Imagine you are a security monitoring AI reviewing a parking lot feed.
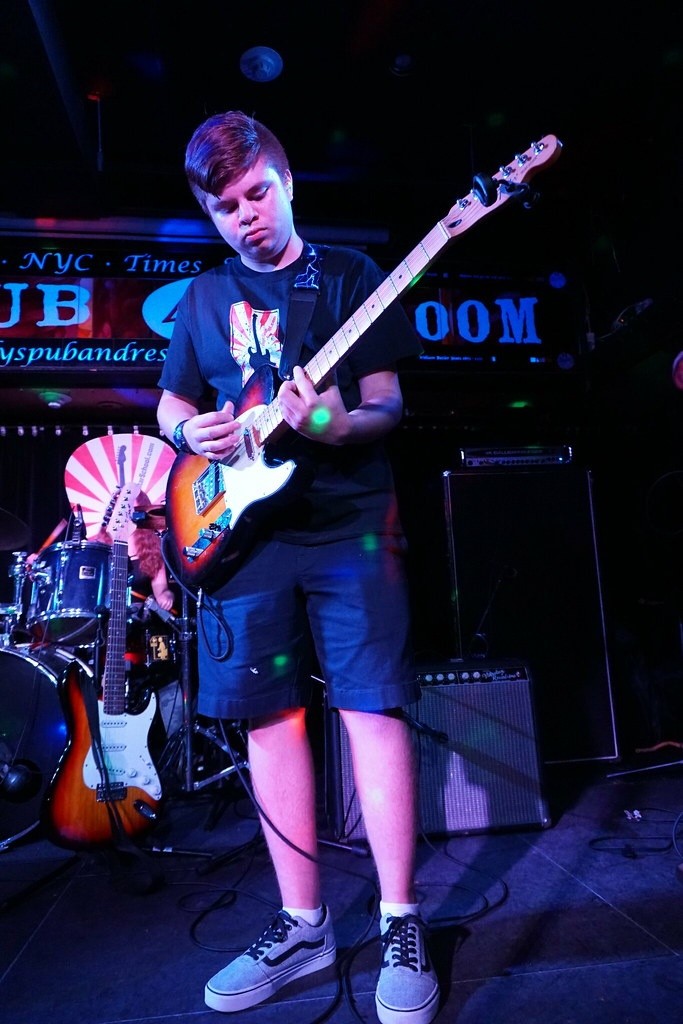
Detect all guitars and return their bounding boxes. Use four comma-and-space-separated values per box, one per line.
35, 482, 166, 855
158, 131, 567, 587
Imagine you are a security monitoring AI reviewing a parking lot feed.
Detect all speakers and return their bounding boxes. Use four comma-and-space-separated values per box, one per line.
442, 468, 619, 765
322, 657, 552, 844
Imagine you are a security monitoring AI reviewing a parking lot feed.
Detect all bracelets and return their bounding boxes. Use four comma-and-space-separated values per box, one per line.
172, 420, 197, 457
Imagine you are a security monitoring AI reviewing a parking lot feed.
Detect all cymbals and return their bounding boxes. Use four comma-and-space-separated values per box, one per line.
0, 508, 32, 551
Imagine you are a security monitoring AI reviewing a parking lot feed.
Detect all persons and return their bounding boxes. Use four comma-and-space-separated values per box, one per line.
156, 112, 441, 1022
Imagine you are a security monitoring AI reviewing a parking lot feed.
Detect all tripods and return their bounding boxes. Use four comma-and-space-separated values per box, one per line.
147, 525, 250, 796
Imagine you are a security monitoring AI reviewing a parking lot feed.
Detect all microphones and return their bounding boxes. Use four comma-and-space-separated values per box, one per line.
77, 504, 86, 539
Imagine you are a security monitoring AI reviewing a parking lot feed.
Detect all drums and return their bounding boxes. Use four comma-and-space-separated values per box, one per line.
0, 641, 98, 844
24, 539, 134, 648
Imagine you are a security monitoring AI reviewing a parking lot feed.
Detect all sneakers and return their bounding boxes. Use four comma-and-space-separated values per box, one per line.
374, 910, 439, 1024
204, 902, 337, 1012
